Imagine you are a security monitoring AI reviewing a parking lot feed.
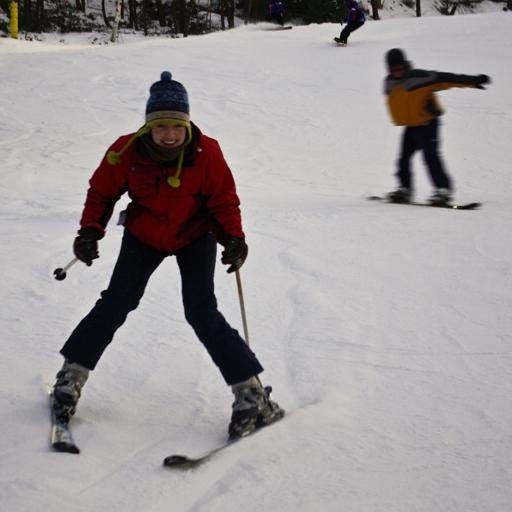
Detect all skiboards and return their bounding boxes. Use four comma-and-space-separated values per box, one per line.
50, 393, 285, 467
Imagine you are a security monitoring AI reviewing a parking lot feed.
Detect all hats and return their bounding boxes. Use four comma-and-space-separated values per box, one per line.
136, 70, 193, 147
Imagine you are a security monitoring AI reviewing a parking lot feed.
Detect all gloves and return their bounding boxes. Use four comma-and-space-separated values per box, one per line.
473, 73, 494, 92
71, 234, 101, 267
220, 239, 248, 274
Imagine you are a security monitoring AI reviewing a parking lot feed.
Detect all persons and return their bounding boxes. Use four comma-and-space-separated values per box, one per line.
385, 48, 491, 203
54, 80, 276, 440
334, 1, 367, 44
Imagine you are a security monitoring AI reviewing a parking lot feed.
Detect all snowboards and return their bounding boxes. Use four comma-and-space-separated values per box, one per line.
334, 37, 346, 46
370, 197, 481, 209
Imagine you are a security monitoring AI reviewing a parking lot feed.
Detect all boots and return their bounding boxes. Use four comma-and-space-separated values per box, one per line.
388, 181, 414, 201
52, 360, 90, 405
426, 187, 456, 202
225, 384, 282, 437
333, 37, 349, 44
